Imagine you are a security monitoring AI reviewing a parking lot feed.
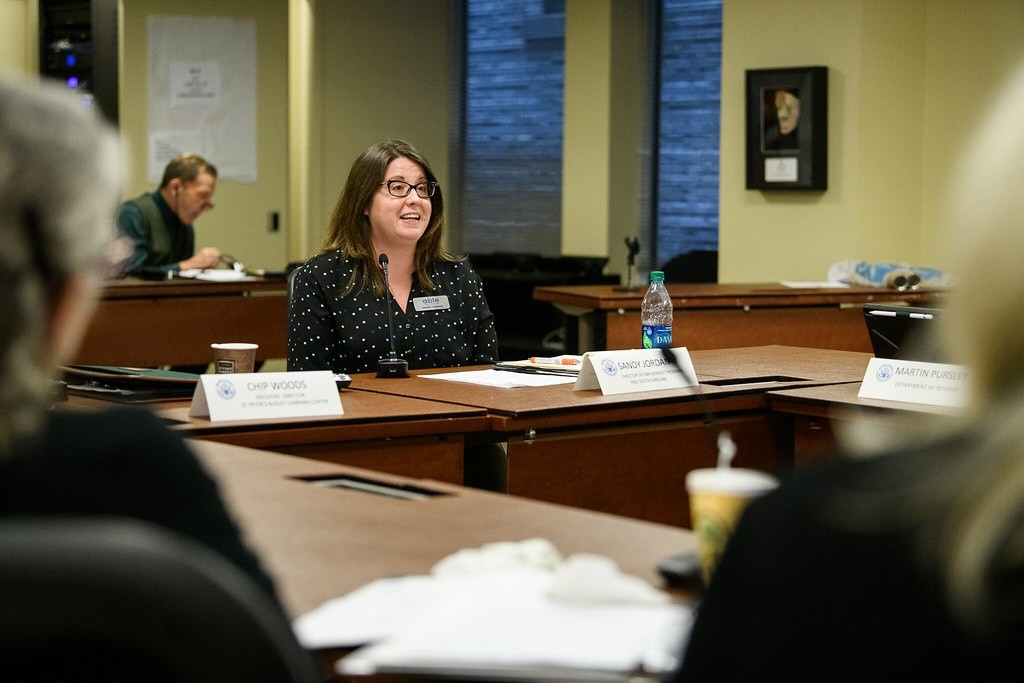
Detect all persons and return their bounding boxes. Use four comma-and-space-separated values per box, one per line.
0, 74, 307, 683
287, 139, 500, 374
667, 67, 1024, 683
118, 153, 222, 273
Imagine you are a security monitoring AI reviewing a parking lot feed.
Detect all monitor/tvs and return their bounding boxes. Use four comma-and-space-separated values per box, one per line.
862, 304, 943, 364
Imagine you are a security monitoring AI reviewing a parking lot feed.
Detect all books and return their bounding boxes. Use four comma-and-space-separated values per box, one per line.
494, 354, 584, 378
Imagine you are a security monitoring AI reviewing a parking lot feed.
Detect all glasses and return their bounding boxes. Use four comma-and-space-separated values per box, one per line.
380, 180, 438, 199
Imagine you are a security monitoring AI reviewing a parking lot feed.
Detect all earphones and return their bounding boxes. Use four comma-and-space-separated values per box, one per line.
174, 187, 178, 192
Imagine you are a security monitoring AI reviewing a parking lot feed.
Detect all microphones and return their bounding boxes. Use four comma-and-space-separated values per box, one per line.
661, 346, 717, 446
374, 253, 411, 378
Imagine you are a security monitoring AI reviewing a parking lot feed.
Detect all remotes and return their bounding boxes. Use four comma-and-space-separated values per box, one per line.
333, 373, 352, 387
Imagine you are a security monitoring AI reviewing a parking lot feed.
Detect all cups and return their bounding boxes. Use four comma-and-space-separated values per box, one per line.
688, 470, 777, 585
212, 343, 258, 373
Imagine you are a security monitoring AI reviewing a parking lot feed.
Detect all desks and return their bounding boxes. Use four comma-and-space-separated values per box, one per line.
63, 343, 986, 539
534, 283, 956, 371
66, 274, 313, 370
181, 436, 762, 683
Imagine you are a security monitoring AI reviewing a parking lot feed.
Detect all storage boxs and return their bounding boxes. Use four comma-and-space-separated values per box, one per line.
745, 66, 829, 192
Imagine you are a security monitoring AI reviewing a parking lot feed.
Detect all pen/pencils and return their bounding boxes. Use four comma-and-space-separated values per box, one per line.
527, 356, 581, 365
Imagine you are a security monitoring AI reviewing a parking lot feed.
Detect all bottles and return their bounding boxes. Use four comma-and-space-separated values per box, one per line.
641, 271, 672, 349
